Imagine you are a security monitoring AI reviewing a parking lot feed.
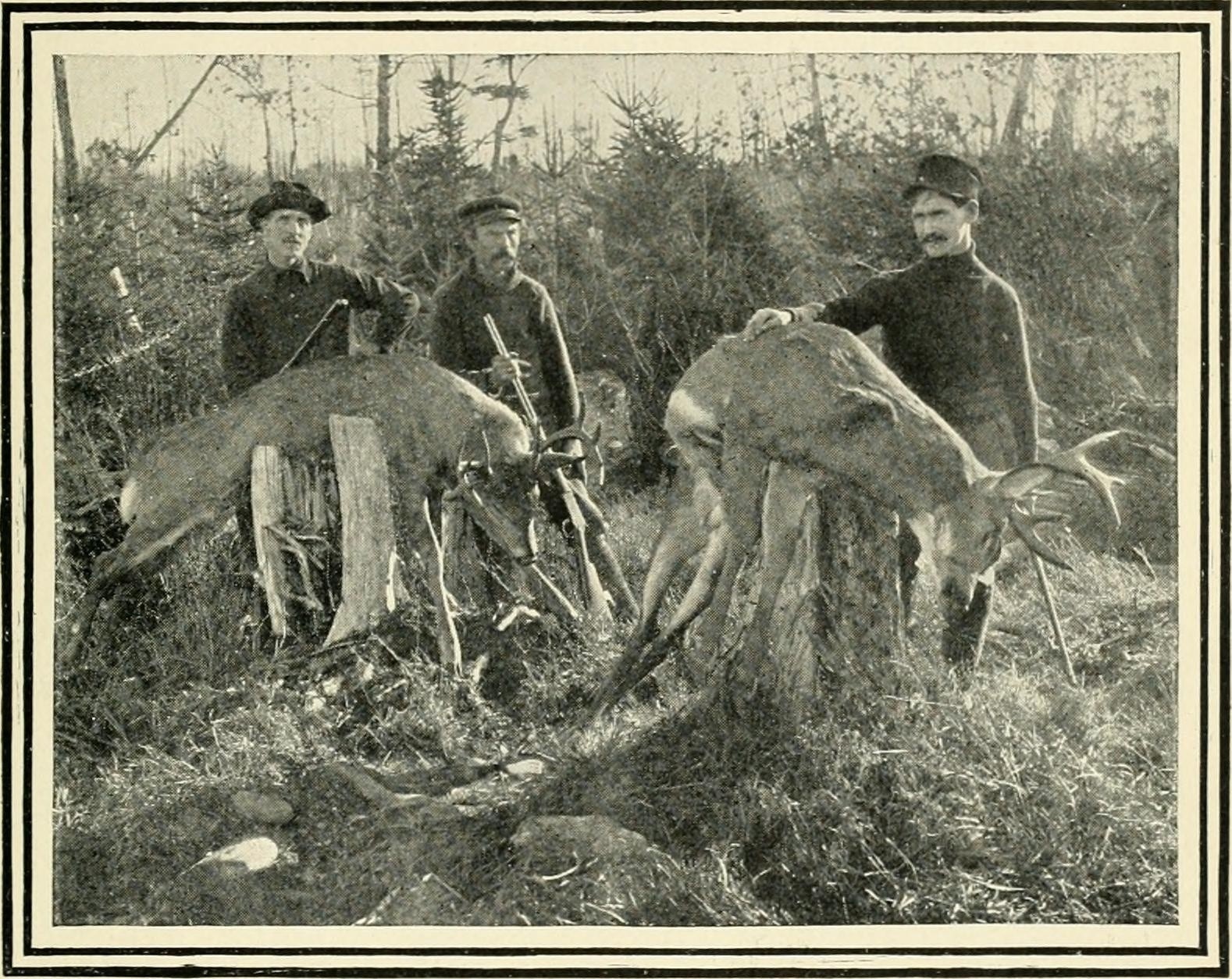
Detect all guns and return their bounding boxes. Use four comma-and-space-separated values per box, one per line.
280, 296, 353, 373
994, 419, 1079, 692
481, 309, 642, 623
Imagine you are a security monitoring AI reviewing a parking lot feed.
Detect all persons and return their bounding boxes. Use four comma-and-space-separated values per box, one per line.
429, 197, 586, 609
742, 155, 1038, 690
221, 179, 421, 648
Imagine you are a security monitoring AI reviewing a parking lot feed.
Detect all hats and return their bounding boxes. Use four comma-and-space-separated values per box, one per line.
248, 179, 333, 230
901, 153, 983, 203
458, 194, 523, 230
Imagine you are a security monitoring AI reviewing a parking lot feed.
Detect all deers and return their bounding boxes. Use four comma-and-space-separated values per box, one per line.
586, 318, 1177, 739
60, 355, 606, 691
